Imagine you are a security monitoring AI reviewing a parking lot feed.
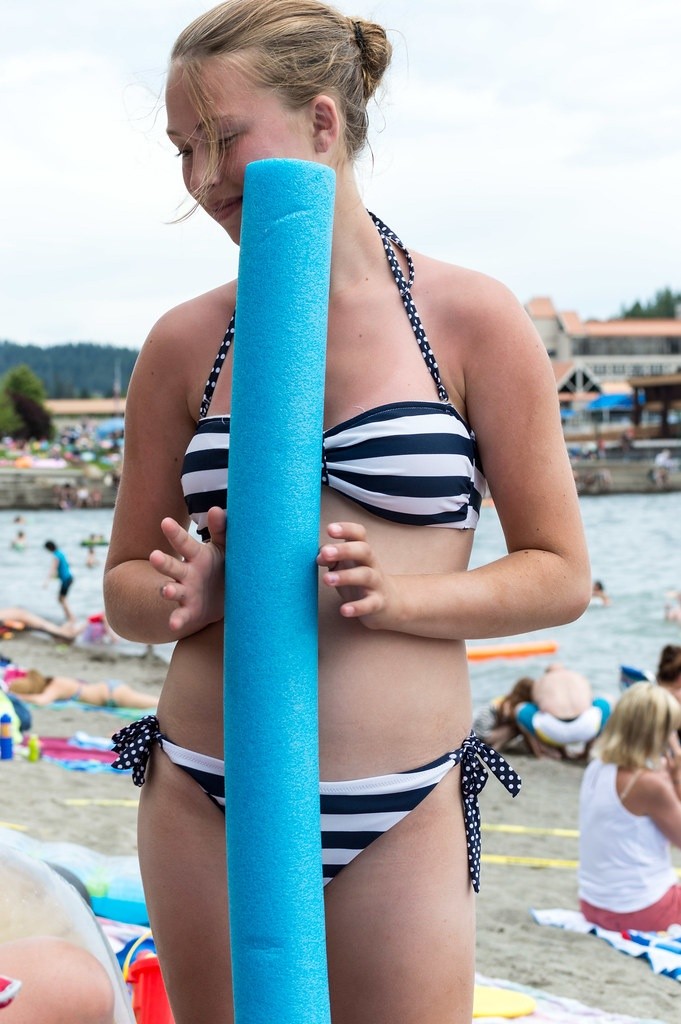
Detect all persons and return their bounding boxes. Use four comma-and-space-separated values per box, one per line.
102, 1, 593, 1023
566, 429, 675, 493
0, 512, 159, 711
0, 935, 115, 1024
0, 417, 121, 514
470, 576, 680, 933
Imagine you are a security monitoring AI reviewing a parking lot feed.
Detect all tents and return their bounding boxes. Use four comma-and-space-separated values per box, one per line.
585, 392, 645, 430
